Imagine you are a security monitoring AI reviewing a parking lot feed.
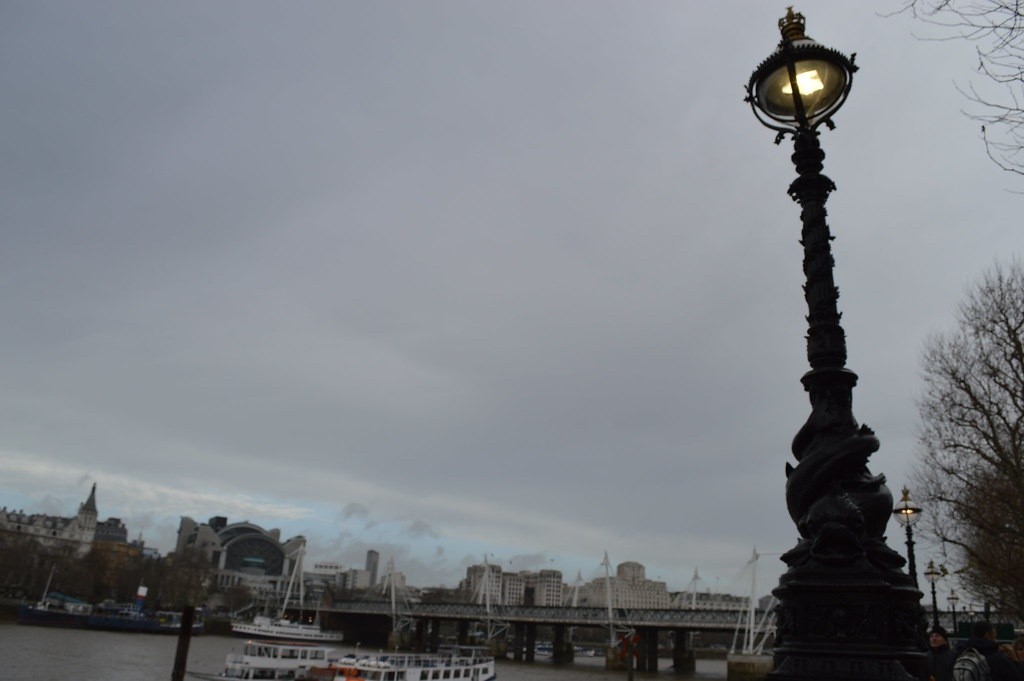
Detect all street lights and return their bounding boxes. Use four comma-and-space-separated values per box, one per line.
925, 559, 945, 635
743, 5, 933, 681
946, 588, 960, 637
891, 484, 923, 592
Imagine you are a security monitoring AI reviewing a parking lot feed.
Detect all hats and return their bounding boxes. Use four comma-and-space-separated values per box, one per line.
929, 626, 948, 639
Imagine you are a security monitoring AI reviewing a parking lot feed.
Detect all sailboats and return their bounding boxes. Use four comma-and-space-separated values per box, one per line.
230, 542, 344, 644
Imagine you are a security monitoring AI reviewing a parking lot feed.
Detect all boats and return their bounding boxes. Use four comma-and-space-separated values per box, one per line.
324, 642, 497, 681
20, 560, 163, 631
183, 638, 344, 681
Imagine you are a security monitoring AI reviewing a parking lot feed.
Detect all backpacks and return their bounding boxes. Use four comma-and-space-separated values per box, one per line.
952, 646, 997, 681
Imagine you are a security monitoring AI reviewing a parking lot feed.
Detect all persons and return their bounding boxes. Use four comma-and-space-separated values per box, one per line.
946, 621, 1024, 681
929, 627, 953, 681
